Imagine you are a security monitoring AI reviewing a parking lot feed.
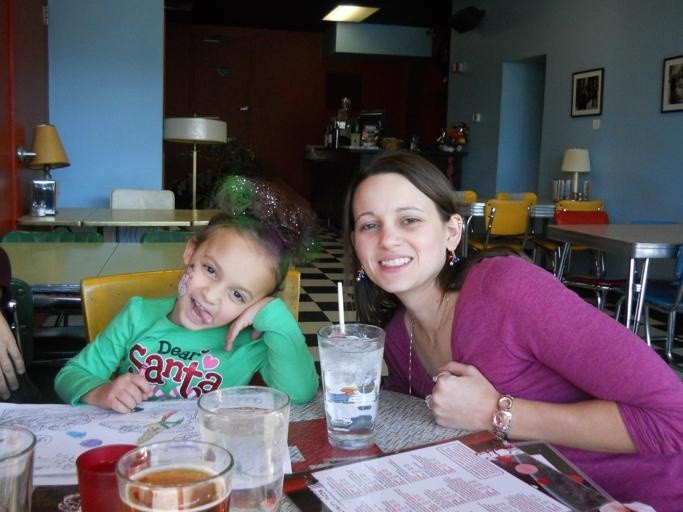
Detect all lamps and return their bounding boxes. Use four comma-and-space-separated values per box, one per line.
556, 145, 594, 198
23, 119, 72, 182
160, 111, 232, 209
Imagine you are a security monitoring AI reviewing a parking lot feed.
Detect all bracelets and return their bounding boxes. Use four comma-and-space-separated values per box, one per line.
491, 394, 514, 441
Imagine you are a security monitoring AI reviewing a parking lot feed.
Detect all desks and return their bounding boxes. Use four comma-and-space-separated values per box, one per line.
303, 145, 474, 230
1, 390, 629, 511
0, 239, 191, 288
2, 207, 234, 244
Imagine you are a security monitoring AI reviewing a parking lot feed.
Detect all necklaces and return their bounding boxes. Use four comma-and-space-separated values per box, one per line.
408, 290, 451, 395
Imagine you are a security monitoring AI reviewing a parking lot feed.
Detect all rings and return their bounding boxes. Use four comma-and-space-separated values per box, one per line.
425, 393, 432, 409
432, 371, 450, 383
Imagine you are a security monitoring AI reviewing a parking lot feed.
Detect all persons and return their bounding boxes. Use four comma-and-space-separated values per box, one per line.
0, 247, 27, 402
342, 149, 682, 512
53, 173, 321, 414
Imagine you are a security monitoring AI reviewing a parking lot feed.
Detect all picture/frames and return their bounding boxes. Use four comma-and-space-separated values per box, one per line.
659, 53, 683, 118
567, 66, 607, 120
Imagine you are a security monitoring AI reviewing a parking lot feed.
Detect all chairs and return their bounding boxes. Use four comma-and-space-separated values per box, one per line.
3, 278, 82, 401
451, 188, 682, 359
110, 188, 175, 209
81, 267, 302, 343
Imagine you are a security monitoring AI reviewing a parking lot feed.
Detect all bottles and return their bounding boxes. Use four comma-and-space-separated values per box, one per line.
552, 177, 570, 201
322, 116, 339, 148
578, 178, 590, 201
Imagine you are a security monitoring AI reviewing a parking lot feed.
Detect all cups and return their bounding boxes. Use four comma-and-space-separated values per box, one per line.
195, 385, 291, 512
0, 424, 36, 511
73, 442, 154, 512
317, 324, 387, 448
118, 442, 234, 512
